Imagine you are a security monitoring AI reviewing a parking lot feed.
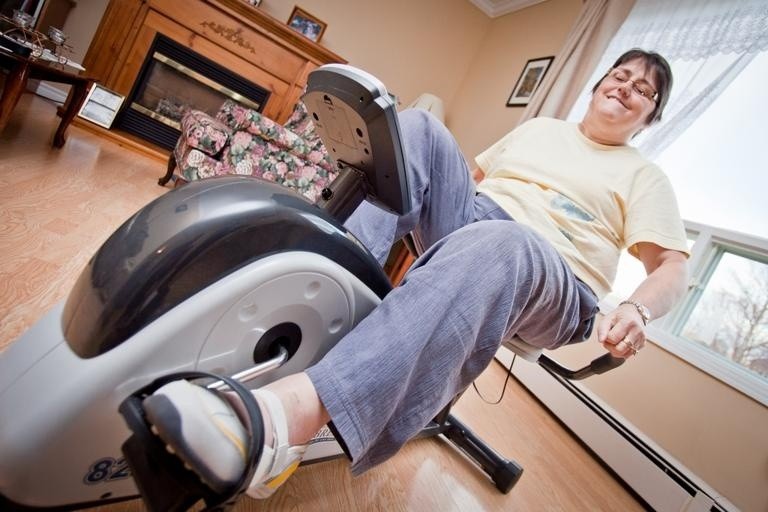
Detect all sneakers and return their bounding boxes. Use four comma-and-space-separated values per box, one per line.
137, 374, 312, 502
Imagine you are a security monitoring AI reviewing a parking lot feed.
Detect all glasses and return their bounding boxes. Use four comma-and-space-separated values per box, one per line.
606, 65, 656, 100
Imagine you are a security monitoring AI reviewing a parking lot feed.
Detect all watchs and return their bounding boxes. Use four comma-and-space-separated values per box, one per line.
618, 300, 651, 326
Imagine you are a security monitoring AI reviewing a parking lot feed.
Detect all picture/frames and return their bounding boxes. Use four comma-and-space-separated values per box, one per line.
285, 5, 329, 42
506, 55, 556, 108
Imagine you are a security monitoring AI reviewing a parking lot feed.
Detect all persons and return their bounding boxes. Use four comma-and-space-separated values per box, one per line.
139, 44, 691, 500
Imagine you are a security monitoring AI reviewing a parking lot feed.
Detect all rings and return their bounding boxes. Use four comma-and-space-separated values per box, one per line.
622, 338, 633, 348
629, 347, 639, 356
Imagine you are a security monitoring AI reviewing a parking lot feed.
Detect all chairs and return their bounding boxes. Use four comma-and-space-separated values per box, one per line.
158, 81, 339, 202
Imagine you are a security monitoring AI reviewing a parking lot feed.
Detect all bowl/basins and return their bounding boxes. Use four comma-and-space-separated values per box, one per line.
13, 8, 35, 29
47, 25, 65, 46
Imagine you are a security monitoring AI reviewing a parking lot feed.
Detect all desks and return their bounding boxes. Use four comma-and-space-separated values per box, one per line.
0, 37, 102, 149
